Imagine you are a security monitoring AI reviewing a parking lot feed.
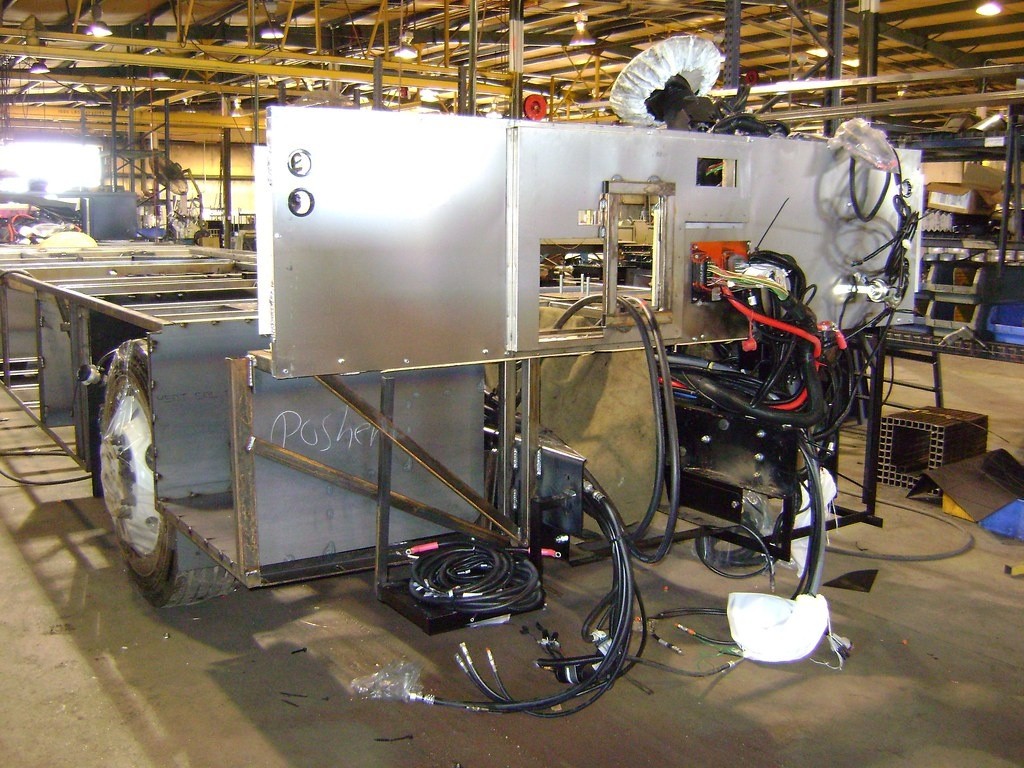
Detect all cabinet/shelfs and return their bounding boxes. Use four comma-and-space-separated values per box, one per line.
884, 136, 1024, 364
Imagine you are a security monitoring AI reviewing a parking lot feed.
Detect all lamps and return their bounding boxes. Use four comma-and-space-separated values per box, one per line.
182, 98, 196, 114
151, 72, 170, 82
84, 4, 113, 37
793, 54, 809, 82
713, 31, 726, 63
30, 58, 50, 74
569, 21, 596, 46
260, 1, 284, 39
230, 98, 246, 117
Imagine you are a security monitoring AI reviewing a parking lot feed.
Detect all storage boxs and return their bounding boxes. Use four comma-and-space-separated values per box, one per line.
926, 163, 1005, 215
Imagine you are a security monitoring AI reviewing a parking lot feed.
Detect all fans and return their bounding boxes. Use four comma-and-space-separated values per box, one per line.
149, 155, 204, 229
